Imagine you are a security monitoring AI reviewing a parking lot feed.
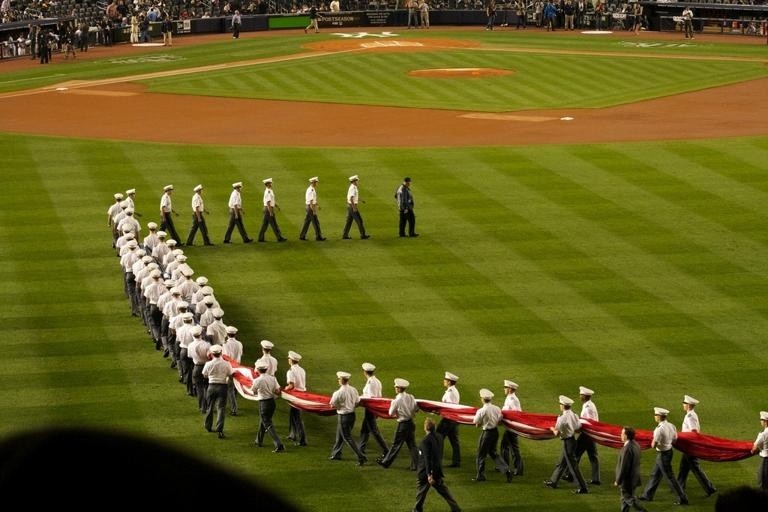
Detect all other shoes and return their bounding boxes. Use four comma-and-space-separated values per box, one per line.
446, 462, 459, 468
543, 480, 557, 489
512, 472, 523, 475
495, 466, 501, 472
704, 489, 717, 498
586, 479, 600, 485
572, 488, 588, 495
255, 436, 308, 453
472, 475, 485, 483
327, 443, 419, 472
637, 495, 653, 501
673, 500, 688, 505
560, 475, 574, 483
505, 471, 513, 483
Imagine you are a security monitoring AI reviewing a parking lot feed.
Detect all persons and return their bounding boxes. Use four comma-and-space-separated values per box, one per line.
752, 410, 768, 490
682, 7, 694, 40
434, 370, 461, 468
542, 386, 601, 494
107, 181, 253, 442
614, 395, 716, 510
253, 339, 308, 452
342, 174, 371, 240
715, 486, 767, 510
178, 1, 340, 39
486, 0, 644, 34
258, 177, 288, 243
299, 176, 327, 241
396, 177, 418, 237
413, 418, 462, 511
329, 362, 390, 465
405, 0, 430, 29
1, 1, 174, 64
375, 377, 420, 471
472, 380, 523, 483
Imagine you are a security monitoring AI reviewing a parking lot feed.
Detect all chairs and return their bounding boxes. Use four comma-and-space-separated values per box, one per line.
1, 1, 223, 48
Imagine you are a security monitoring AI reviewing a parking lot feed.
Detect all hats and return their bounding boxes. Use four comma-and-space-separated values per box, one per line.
255, 358, 269, 370
479, 388, 494, 401
444, 371, 459, 382
262, 177, 273, 185
260, 339, 275, 350
653, 406, 670, 417
681, 394, 700, 405
760, 410, 768, 421
558, 394, 575, 407
578, 386, 595, 397
308, 176, 318, 183
348, 174, 360, 182
287, 350, 302, 362
393, 377, 410, 389
503, 379, 519, 390
336, 370, 352, 380
361, 362, 376, 372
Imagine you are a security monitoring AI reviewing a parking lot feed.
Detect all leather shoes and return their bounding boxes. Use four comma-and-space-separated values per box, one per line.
257, 233, 419, 242
114, 179, 253, 439
